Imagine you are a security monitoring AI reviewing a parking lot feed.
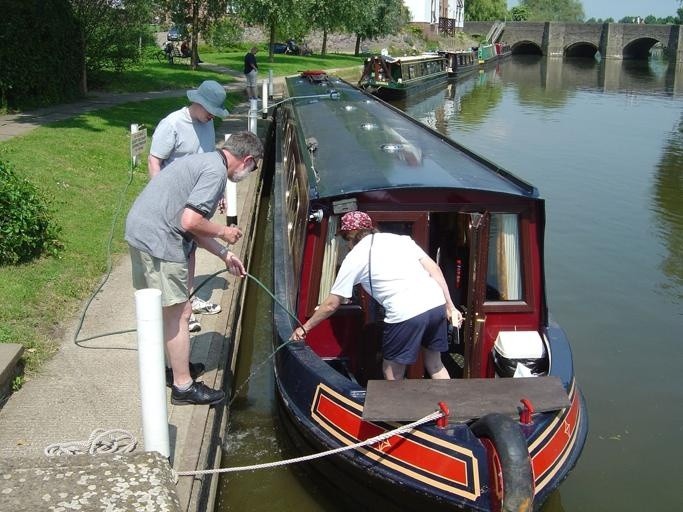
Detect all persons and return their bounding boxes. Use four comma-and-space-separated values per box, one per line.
146, 79, 228, 331
289, 211, 462, 380
125, 131, 265, 404
181, 40, 204, 63
244, 47, 261, 100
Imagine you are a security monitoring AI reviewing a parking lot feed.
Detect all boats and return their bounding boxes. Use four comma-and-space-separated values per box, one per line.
275, 72, 590, 512
357, 40, 513, 98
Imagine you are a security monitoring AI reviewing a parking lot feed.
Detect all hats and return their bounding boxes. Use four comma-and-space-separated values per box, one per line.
334, 211, 374, 238
186, 80, 230, 119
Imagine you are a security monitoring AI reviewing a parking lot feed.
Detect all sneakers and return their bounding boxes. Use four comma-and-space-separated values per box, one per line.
191, 296, 223, 315
188, 313, 202, 332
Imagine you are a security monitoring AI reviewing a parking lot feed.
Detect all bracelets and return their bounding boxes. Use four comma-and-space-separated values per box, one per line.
220, 248, 228, 255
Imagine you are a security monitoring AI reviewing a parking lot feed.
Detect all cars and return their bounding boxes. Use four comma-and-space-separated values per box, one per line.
273, 43, 304, 54
167, 25, 189, 41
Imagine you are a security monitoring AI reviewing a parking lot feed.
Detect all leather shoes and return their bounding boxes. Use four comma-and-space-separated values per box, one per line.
165, 361, 205, 386
171, 383, 225, 405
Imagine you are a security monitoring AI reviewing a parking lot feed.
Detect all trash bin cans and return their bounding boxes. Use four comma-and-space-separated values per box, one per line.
492, 330, 549, 378
